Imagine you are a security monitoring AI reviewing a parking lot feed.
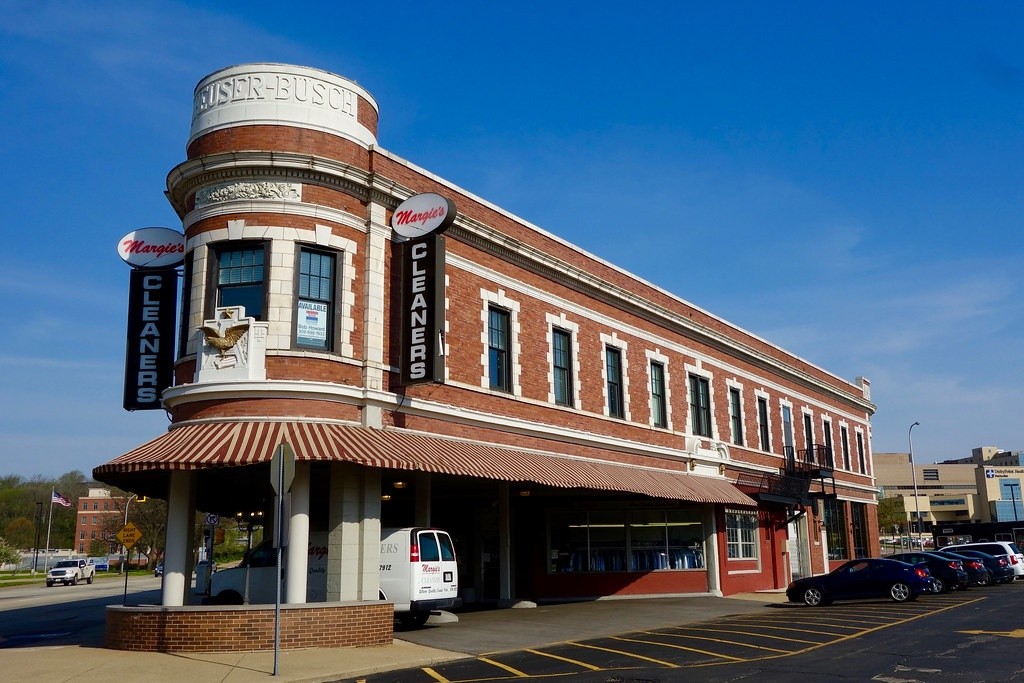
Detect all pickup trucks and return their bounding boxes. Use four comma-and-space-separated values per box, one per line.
46, 559, 96, 585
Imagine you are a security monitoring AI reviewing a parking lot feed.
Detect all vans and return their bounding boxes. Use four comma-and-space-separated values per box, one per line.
202, 527, 464, 630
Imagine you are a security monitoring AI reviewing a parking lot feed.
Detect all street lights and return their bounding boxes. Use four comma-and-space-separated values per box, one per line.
235, 510, 265, 604
909, 421, 924, 552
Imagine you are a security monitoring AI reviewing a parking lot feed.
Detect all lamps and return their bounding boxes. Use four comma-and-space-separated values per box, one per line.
691, 458, 697, 467
520, 489, 530, 496
393, 481, 407, 488
381, 494, 391, 500
818, 521, 827, 527
720, 463, 726, 472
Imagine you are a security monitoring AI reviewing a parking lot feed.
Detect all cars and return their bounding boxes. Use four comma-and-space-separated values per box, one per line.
155, 556, 165, 577
885, 553, 965, 597
880, 537, 934, 547
195, 559, 216, 573
933, 552, 988, 589
955, 550, 1010, 586
785, 558, 931, 605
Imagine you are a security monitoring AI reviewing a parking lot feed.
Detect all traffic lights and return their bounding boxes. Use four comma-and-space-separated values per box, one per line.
137, 494, 146, 503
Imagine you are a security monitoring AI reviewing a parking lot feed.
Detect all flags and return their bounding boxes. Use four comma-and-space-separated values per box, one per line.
52, 490, 72, 507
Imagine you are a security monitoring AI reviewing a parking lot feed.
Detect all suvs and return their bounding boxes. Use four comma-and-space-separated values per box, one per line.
940, 542, 1024, 582
